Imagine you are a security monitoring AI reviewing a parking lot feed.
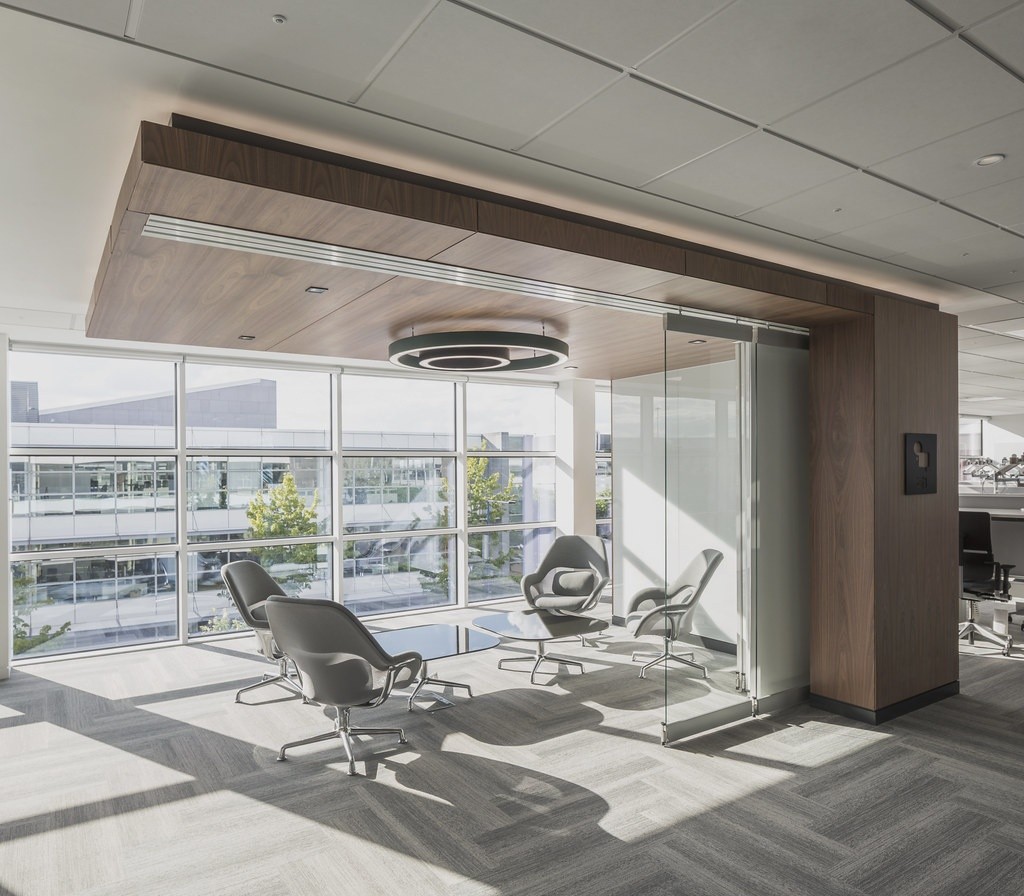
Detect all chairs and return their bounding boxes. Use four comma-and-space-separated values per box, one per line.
521, 535, 610, 646
221, 560, 309, 705
265, 596, 422, 775
959, 511, 1016, 656
626, 549, 724, 679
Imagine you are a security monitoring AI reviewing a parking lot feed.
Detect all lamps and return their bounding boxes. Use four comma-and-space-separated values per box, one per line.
388, 326, 569, 373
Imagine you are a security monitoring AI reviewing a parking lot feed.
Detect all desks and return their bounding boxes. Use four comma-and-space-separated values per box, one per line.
472, 609, 610, 684
370, 623, 500, 712
